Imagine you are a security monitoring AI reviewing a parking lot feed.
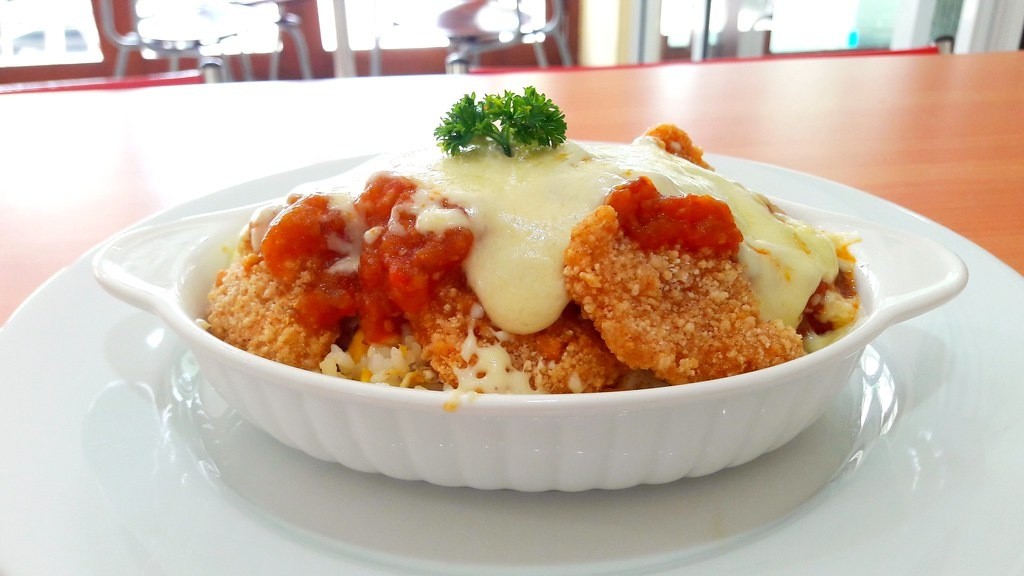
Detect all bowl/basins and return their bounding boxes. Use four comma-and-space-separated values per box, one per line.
91, 193, 970, 494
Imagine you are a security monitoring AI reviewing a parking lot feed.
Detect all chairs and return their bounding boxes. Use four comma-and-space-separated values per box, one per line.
99, 0, 577, 81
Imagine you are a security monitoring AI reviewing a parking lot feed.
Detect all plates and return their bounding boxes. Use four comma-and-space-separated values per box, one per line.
0, 139, 1024, 576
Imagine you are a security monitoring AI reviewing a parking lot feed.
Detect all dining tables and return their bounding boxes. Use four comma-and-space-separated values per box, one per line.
0, 47, 1024, 328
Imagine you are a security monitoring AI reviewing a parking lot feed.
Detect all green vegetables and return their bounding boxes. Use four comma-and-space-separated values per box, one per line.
432, 87, 569, 157
